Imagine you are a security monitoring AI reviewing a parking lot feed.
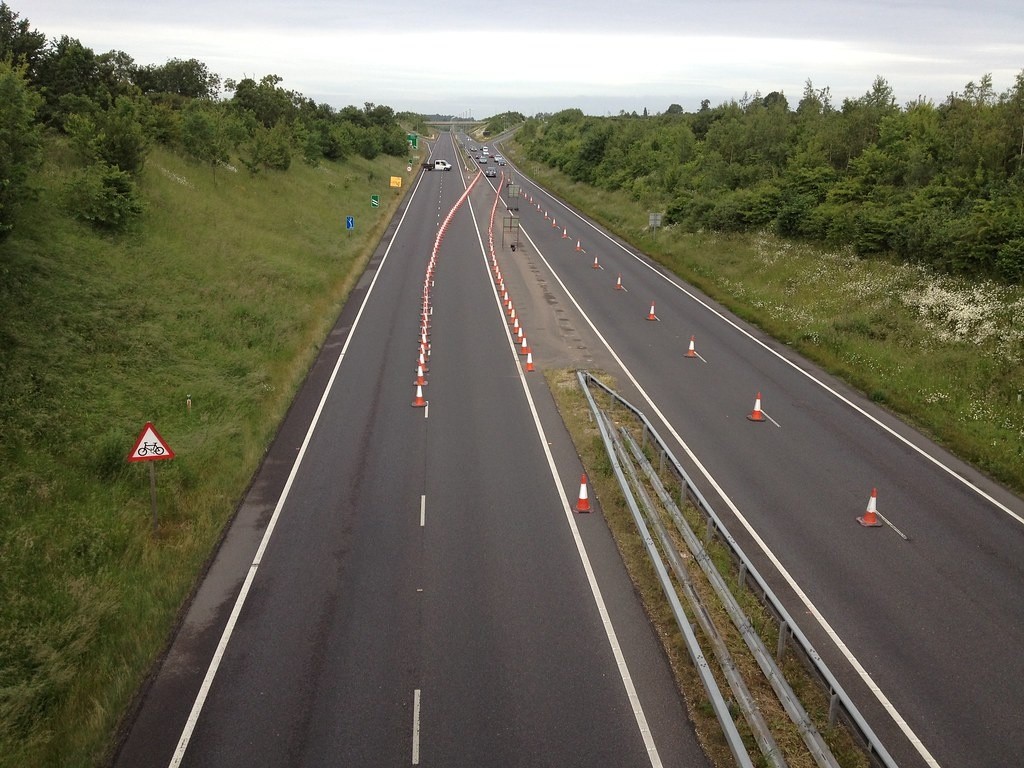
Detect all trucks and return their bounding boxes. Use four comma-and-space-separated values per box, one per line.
420, 160, 453, 171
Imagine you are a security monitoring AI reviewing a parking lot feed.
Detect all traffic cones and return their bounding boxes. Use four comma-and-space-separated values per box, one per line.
591, 256, 601, 269
415, 347, 430, 374
413, 363, 429, 386
646, 300, 658, 321
416, 339, 430, 362
856, 488, 883, 527
524, 347, 536, 371
747, 392, 766, 422
505, 296, 514, 316
507, 305, 517, 324
517, 331, 532, 355
414, 168, 568, 329
682, 335, 697, 358
572, 474, 595, 514
410, 376, 428, 408
417, 328, 431, 351
500, 284, 506, 297
574, 239, 581, 252
502, 288, 510, 307
612, 272, 624, 291
513, 323, 526, 344
512, 313, 520, 335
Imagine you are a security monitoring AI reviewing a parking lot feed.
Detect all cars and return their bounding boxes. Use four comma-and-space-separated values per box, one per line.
470, 146, 507, 167
484, 166, 497, 177
